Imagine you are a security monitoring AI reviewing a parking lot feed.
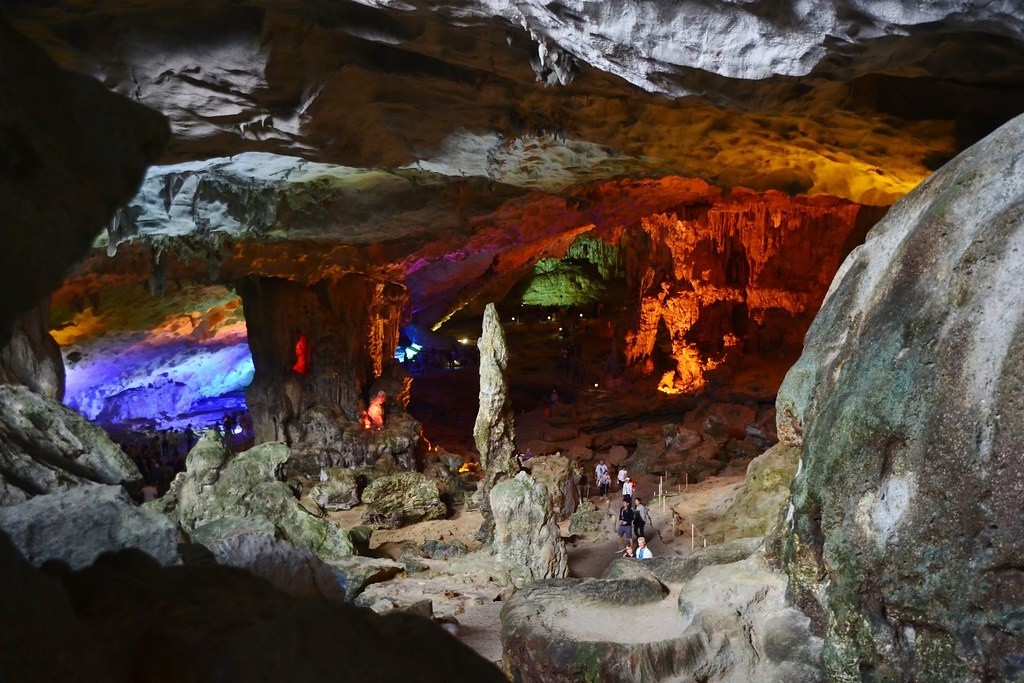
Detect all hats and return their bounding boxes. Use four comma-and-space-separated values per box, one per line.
625, 478, 631, 481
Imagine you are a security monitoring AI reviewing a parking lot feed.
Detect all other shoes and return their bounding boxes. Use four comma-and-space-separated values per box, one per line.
614, 546, 626, 553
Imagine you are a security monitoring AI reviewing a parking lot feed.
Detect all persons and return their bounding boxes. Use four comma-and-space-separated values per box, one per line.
636, 537, 652, 558
576, 456, 582, 466
119, 426, 179, 474
517, 305, 581, 357
614, 499, 634, 553
623, 476, 635, 501
214, 411, 252, 441
458, 457, 478, 473
617, 465, 628, 493
523, 448, 532, 460
599, 471, 612, 502
550, 390, 559, 413
184, 424, 198, 451
624, 545, 636, 558
633, 497, 647, 538
403, 342, 476, 369
595, 460, 607, 487
